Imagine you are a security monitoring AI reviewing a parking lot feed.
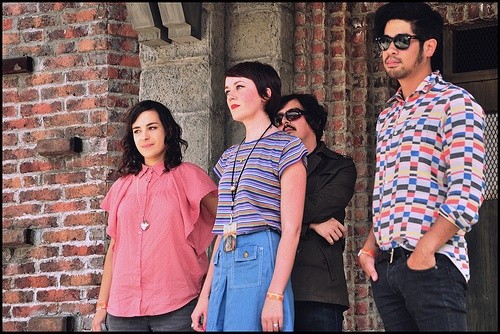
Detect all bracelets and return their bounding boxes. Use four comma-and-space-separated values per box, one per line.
266, 292, 284, 301
96, 305, 107, 310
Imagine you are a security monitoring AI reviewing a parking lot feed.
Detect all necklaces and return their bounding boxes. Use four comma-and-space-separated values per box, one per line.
136, 174, 164, 232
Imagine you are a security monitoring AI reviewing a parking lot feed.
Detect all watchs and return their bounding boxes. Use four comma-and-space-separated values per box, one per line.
357, 248, 376, 258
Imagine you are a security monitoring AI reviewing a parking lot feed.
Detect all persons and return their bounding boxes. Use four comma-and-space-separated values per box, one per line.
191, 60, 358, 333
357, 0, 487, 334
90, 99, 218, 333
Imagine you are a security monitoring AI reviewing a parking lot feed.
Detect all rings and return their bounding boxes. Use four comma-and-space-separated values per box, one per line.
91, 329, 93, 332
273, 324, 278, 327
190, 323, 194, 328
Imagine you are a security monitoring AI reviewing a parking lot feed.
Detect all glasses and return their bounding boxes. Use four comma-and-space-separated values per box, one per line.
374, 34, 419, 50
274, 108, 306, 127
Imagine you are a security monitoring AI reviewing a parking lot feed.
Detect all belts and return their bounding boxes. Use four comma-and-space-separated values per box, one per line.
378, 247, 413, 263
